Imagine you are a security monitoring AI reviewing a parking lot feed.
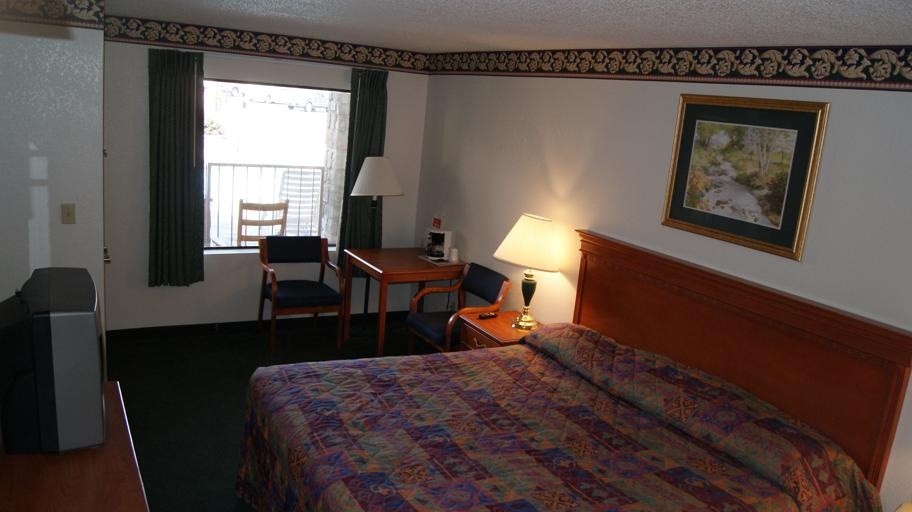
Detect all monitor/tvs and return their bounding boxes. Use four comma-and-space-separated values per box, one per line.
0, 267, 107, 455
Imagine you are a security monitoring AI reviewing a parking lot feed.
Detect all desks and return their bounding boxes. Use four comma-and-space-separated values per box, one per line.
0, 380, 151, 512
343, 248, 466, 357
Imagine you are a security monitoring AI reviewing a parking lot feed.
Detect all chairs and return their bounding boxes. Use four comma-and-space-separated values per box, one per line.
258, 236, 346, 358
212, 199, 289, 246
405, 262, 512, 356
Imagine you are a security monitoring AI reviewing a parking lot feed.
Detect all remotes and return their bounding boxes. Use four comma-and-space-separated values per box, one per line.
480, 312, 496, 318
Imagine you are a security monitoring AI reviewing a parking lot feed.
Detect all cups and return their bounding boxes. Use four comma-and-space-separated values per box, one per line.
449, 248, 459, 263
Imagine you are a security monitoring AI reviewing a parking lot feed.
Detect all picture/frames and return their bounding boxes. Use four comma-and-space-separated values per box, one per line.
660, 93, 832, 263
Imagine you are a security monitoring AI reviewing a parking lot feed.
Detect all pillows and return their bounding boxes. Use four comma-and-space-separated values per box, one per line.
518, 323, 866, 511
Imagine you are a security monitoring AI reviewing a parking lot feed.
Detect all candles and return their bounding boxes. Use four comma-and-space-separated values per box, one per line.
449, 245, 459, 263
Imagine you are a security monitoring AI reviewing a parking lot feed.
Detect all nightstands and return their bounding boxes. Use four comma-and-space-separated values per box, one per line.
457, 310, 546, 351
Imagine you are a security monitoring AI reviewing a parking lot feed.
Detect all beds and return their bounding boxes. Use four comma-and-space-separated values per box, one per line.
235, 229, 912, 512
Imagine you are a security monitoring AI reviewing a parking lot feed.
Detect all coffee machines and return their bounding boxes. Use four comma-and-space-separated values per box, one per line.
425, 230, 453, 260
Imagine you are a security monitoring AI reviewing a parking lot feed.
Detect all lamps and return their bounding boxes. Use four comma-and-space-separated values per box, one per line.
492, 213, 561, 330
350, 156, 406, 320
